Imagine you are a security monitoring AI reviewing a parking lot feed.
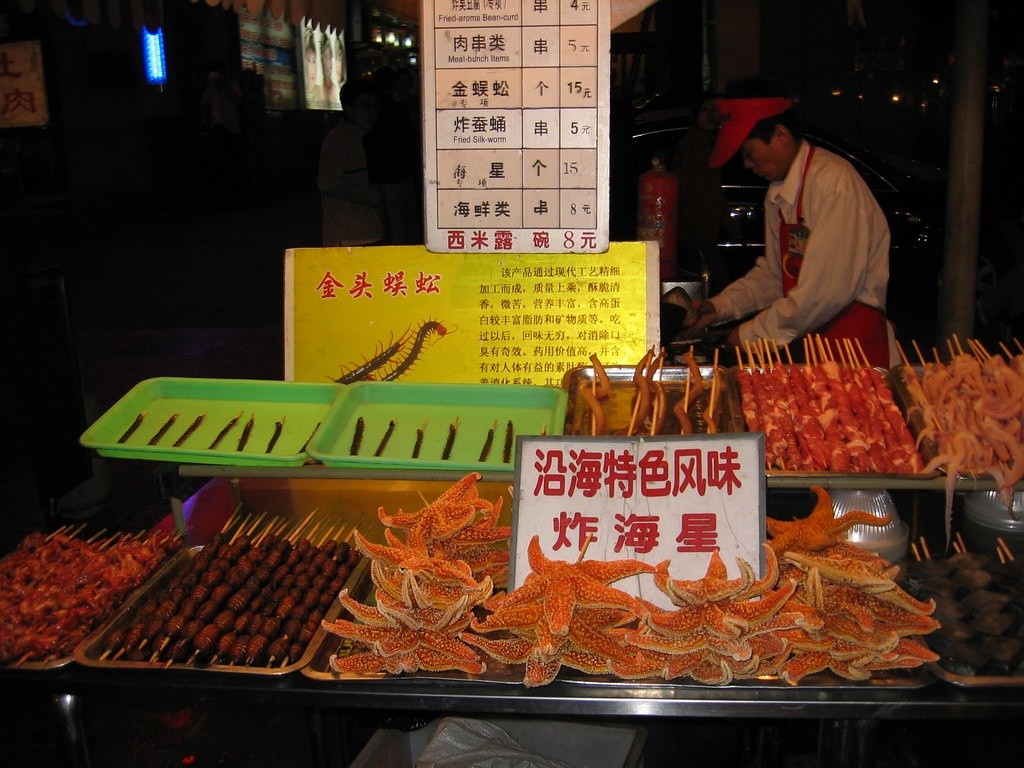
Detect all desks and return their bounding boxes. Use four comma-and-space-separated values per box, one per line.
0, 482, 1024, 768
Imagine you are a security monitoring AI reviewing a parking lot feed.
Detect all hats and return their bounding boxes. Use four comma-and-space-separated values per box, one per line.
710, 98, 791, 168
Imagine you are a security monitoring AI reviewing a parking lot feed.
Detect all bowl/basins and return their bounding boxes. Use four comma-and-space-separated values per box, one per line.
827, 490, 911, 563
963, 488, 1024, 532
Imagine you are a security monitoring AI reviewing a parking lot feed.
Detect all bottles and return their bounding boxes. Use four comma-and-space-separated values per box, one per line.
639, 156, 676, 280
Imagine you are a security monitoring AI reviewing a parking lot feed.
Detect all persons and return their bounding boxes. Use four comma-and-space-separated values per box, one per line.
364, 64, 422, 246
201, 60, 246, 207
683, 97, 890, 367
677, 90, 731, 293
318, 80, 389, 245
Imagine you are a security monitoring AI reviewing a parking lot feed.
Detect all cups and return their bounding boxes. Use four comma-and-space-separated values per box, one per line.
662, 287, 702, 332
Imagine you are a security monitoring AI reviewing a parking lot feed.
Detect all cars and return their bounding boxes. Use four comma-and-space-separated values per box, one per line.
627, 117, 1005, 310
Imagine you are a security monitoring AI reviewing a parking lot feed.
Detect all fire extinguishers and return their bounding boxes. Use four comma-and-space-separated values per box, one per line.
636, 151, 679, 283
